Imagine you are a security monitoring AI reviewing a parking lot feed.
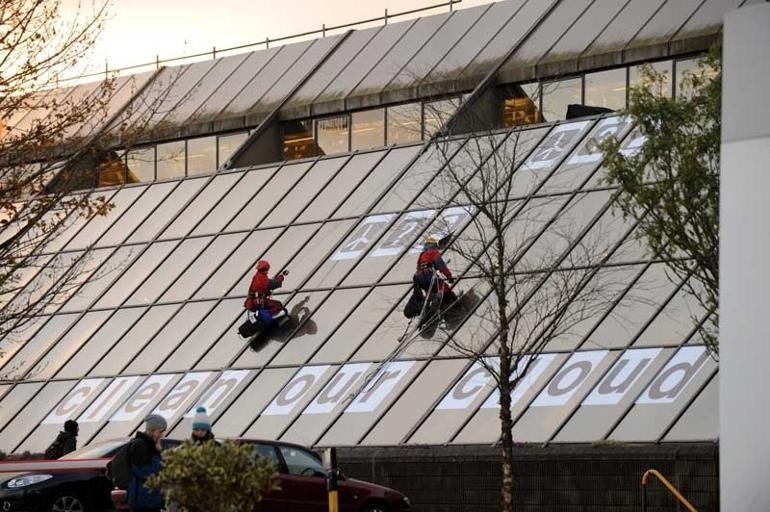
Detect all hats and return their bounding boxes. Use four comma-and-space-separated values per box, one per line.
143, 412, 169, 430
191, 406, 212, 430
63, 419, 77, 431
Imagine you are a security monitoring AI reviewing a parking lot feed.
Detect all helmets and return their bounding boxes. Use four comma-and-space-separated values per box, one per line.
423, 237, 438, 244
255, 259, 271, 270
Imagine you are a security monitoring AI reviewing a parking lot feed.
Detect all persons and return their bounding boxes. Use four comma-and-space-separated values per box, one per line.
183, 406, 222, 450
45, 419, 80, 462
240, 256, 290, 328
416, 236, 455, 300
123, 412, 169, 510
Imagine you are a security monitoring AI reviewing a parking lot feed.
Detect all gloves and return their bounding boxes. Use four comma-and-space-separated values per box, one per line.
448, 278, 454, 283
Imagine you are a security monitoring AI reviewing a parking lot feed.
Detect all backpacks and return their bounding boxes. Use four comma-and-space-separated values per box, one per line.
44, 435, 73, 458
104, 437, 149, 490
